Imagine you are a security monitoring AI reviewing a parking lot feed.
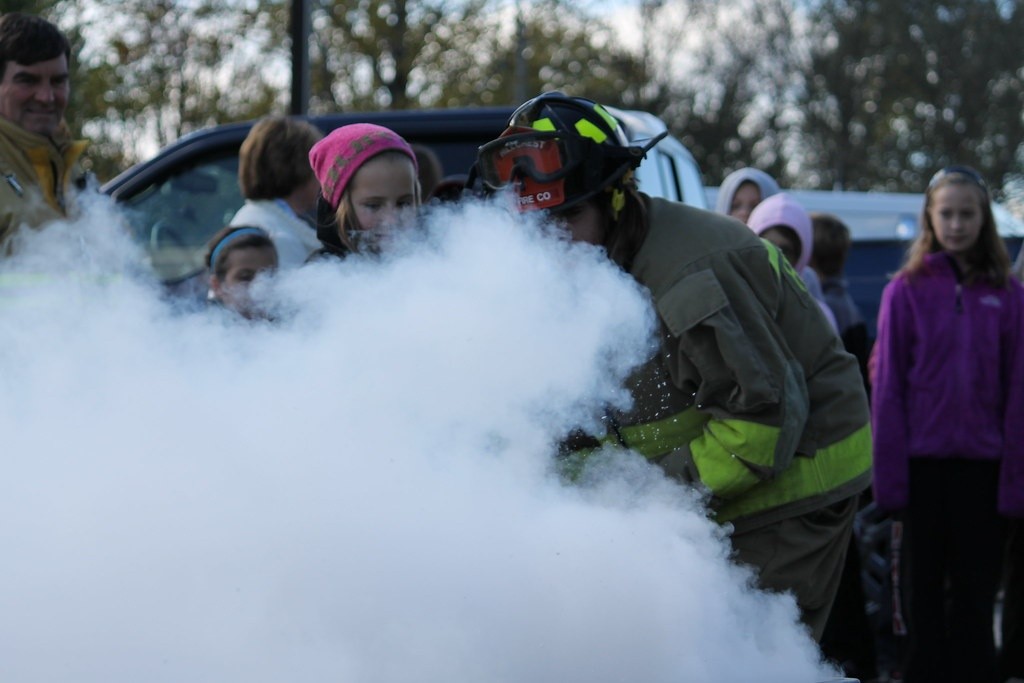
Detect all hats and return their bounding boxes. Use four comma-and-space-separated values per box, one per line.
716, 167, 779, 215
748, 191, 811, 274
307, 123, 418, 207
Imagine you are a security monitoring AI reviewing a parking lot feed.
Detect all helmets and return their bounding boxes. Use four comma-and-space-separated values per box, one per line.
489, 91, 639, 220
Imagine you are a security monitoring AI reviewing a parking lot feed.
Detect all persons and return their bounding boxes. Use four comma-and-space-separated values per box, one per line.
714, 167, 899, 683
230, 118, 326, 264
305, 123, 424, 271
194, 227, 279, 318
468, 90, 872, 648
0, 16, 97, 257
872, 167, 1024, 683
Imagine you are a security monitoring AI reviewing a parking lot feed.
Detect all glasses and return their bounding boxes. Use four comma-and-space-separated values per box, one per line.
476, 131, 596, 191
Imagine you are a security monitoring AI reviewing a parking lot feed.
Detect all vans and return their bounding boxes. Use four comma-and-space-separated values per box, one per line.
94, 102, 710, 315
705, 187, 1024, 385
